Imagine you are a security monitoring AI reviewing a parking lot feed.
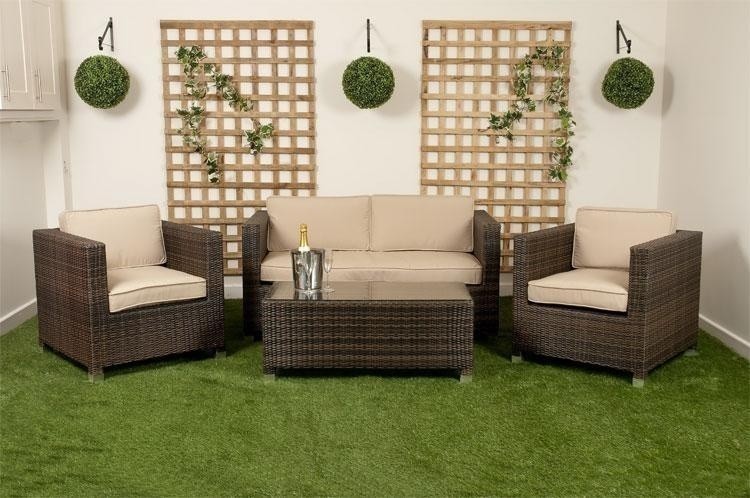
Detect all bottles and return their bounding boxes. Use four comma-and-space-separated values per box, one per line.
298, 223, 311, 251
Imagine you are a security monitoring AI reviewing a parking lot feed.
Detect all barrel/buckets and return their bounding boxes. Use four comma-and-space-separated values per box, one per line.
292, 246, 324, 289
293, 288, 323, 301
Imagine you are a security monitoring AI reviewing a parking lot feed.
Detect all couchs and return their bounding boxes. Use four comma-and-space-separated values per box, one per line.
240, 195, 502, 352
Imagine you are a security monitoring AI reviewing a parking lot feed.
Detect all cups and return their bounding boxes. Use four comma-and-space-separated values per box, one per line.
320, 247, 335, 293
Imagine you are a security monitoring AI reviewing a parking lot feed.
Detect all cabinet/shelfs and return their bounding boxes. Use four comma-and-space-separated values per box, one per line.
0, 0, 67, 121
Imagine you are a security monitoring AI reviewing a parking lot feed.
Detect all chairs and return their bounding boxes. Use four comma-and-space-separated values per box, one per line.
32, 204, 227, 384
511, 207, 703, 388
